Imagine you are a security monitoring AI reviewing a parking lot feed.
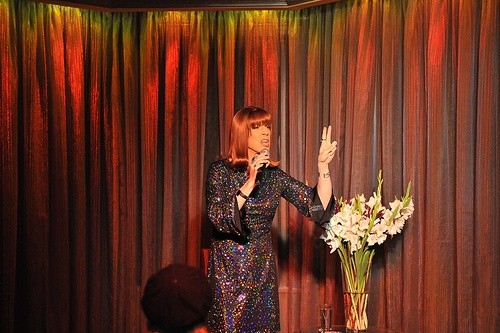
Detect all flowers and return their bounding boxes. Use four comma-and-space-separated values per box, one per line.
318, 169, 416, 330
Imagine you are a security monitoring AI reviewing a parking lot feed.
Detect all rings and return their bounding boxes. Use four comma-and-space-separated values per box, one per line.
320, 137, 327, 143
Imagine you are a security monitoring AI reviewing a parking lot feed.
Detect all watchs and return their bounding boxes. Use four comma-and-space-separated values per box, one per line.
317, 171, 331, 179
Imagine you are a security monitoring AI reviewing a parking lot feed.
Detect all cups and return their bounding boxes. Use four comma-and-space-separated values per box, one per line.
320, 303, 333, 330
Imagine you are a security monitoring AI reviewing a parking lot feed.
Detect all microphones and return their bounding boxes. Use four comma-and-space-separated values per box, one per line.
257, 148, 270, 186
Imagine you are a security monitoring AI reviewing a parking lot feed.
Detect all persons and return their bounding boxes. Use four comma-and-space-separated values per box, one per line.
140, 262, 217, 333
200, 105, 338, 333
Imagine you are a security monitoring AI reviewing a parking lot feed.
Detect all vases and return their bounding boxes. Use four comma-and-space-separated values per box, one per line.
340, 262, 371, 333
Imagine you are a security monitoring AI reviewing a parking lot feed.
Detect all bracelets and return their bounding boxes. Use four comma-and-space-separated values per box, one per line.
235, 190, 250, 200
